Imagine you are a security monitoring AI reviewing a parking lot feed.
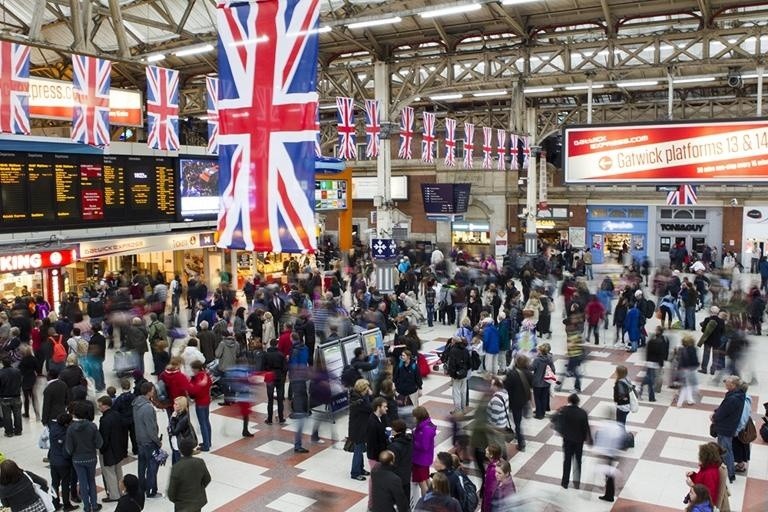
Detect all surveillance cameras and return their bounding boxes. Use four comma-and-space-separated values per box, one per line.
53, 234, 67, 241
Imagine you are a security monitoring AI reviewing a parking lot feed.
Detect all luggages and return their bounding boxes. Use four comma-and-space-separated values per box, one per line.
204, 359, 228, 398
253, 349, 267, 371
112, 347, 143, 378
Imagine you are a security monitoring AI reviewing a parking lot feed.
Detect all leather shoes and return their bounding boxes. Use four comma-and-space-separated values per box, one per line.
312, 438, 325, 444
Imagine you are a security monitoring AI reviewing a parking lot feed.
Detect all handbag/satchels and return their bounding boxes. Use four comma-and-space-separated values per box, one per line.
453, 369, 467, 379
544, 365, 557, 384
669, 370, 687, 389
522, 400, 533, 419
663, 297, 676, 303
523, 309, 534, 316
149, 324, 162, 342
738, 416, 757, 444
710, 424, 718, 438
344, 437, 367, 453
72, 337, 89, 357
248, 336, 262, 351
22, 471, 56, 512
438, 291, 448, 310
657, 310, 663, 319
504, 427, 514, 444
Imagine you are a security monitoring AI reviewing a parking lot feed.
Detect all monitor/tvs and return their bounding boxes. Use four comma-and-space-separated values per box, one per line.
179, 153, 223, 220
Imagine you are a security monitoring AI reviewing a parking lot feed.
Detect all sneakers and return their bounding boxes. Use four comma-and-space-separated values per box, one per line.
264, 416, 287, 426
4, 429, 22, 437
599, 495, 615, 503
560, 480, 581, 489
533, 410, 545, 419
242, 430, 254, 438
351, 469, 370, 481
697, 367, 715, 375
516, 445, 525, 452
146, 492, 163, 499
294, 448, 309, 454
52, 487, 120, 512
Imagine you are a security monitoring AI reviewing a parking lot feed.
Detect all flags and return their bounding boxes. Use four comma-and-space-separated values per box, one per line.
400, 107, 528, 170
336, 96, 359, 160
365, 99, 380, 159
217, 0, 321, 252
666, 185, 698, 204
206, 76, 218, 154
146, 66, 181, 153
71, 55, 112, 146
2, 39, 30, 138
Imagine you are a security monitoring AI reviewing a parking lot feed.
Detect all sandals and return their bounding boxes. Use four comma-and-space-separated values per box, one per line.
734, 463, 746, 472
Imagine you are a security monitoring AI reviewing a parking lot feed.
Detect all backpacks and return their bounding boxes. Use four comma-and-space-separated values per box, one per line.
416, 353, 430, 377
486, 260, 496, 272
305, 297, 313, 310
48, 334, 68, 364
36, 303, 49, 320
461, 480, 479, 512
628, 385, 640, 413
176, 421, 198, 457
468, 349, 481, 371
511, 301, 524, 322
511, 316, 519, 333
150, 379, 174, 409
645, 300, 655, 319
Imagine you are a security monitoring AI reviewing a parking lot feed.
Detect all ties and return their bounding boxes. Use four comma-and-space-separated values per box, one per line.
275, 296, 279, 311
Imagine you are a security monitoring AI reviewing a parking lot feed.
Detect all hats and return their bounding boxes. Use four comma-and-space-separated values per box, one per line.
270, 339, 279, 347
634, 290, 642, 297
67, 353, 77, 366
